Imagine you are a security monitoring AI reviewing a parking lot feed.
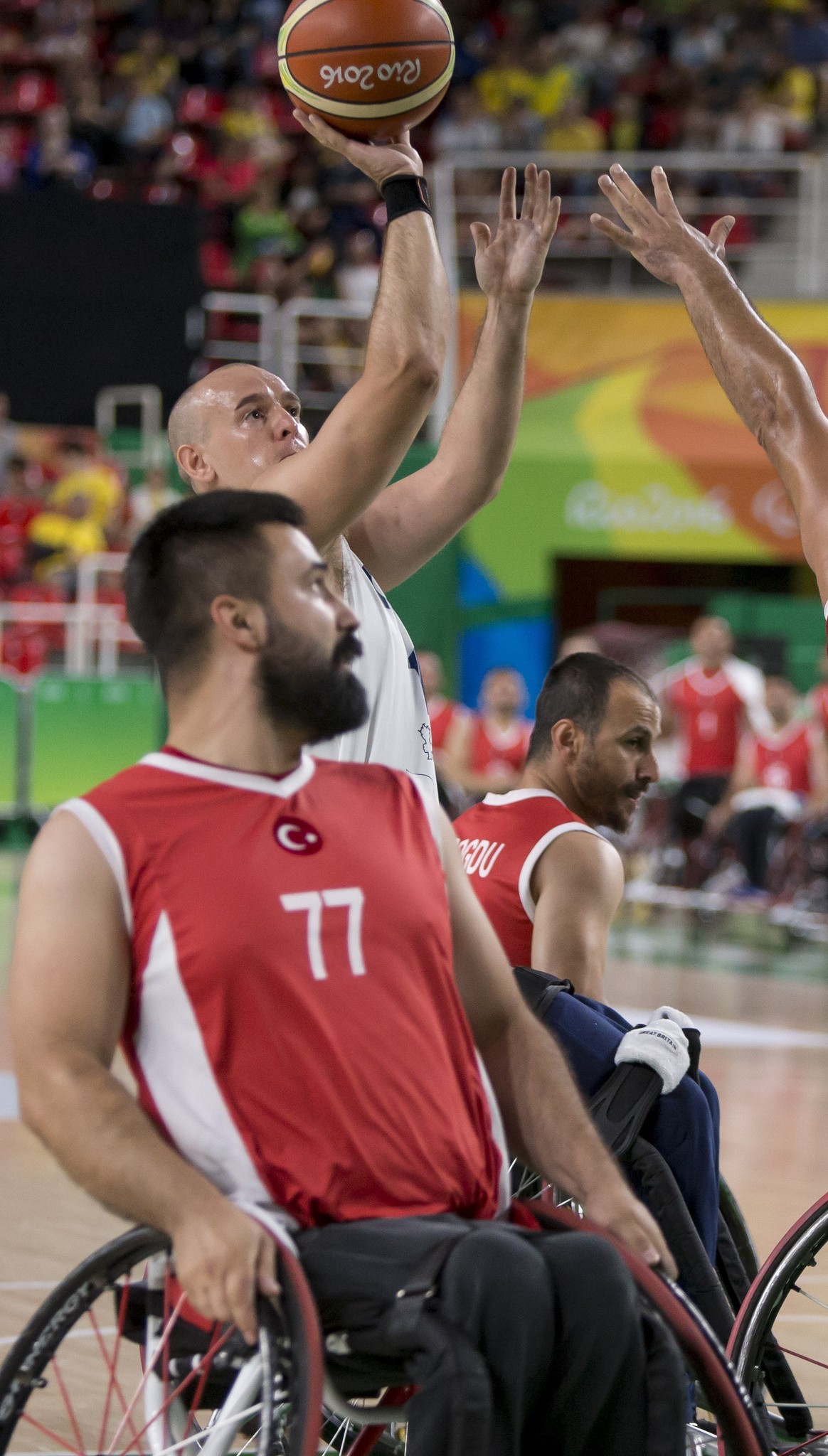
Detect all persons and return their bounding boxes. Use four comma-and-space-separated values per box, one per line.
445, 647, 663, 1005
0, 1, 826, 936
0, 488, 734, 1455
155, 1, 562, 843
588, 163, 828, 649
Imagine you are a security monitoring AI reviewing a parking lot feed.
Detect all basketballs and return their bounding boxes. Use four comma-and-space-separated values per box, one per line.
274, 0, 456, 136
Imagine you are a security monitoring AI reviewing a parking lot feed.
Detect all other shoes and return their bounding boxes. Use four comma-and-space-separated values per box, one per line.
771, 903, 828, 932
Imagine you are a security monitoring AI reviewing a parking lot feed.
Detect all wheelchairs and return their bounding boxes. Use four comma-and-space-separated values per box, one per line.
320, 1152, 586, 1456
714, 1193, 828, 1456
0, 1200, 771, 1456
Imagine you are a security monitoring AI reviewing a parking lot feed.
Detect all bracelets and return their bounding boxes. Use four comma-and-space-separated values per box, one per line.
381, 174, 434, 224
